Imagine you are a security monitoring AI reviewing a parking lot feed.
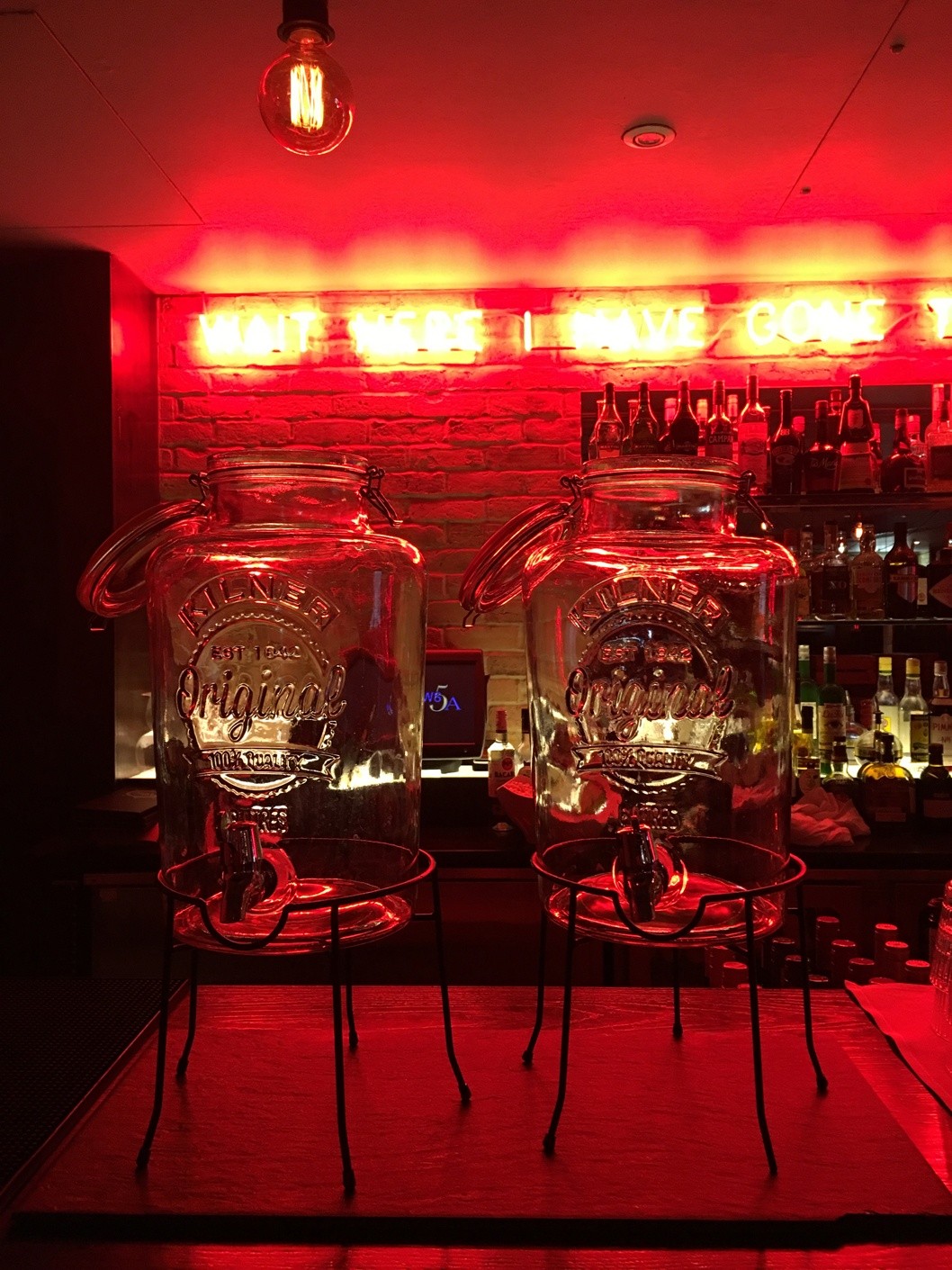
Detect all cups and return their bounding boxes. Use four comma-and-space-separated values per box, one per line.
930, 880, 952, 1043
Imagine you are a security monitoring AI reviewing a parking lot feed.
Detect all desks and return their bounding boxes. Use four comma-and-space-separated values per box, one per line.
1, 977, 952, 1270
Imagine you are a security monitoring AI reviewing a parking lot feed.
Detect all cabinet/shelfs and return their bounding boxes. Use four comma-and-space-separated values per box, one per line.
733, 486, 951, 856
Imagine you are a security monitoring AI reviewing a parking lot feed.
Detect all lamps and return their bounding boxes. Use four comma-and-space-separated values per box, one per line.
258, 0, 356, 156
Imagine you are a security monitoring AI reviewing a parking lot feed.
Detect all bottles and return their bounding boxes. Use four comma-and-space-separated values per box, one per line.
761, 514, 952, 621
789, 643, 952, 836
687, 897, 940, 990
461, 454, 804, 936
585, 365, 952, 500
75, 451, 426, 952
488, 709, 515, 798
